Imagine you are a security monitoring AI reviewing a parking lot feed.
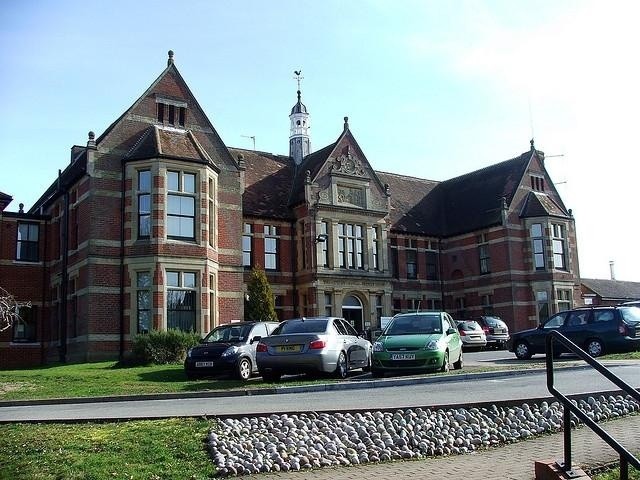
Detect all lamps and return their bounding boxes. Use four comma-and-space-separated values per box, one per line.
315, 234, 329, 245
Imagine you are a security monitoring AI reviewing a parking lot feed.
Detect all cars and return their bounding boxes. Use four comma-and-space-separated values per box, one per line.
254, 315, 373, 378
370, 308, 467, 374
184, 320, 282, 380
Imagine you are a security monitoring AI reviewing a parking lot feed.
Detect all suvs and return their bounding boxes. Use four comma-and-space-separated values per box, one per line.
451, 313, 512, 351
510, 304, 640, 359
